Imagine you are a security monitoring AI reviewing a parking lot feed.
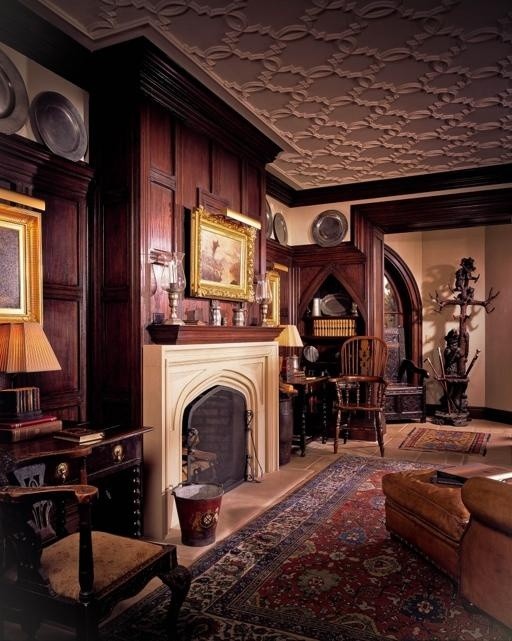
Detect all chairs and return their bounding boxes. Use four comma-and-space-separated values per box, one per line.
329, 336, 388, 457
0, 484, 190, 641
384, 328, 430, 422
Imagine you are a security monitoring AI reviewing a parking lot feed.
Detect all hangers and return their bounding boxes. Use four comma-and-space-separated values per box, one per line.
424, 258, 500, 426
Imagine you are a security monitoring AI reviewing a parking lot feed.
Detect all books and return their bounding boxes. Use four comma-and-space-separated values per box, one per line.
430, 459, 512, 485
313, 319, 357, 337
0, 385, 63, 441
51, 426, 106, 444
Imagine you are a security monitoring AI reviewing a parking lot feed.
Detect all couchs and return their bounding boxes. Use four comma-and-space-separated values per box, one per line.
382, 462, 512, 635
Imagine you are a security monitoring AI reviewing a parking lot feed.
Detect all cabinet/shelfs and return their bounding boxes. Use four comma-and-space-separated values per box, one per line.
143, 342, 278, 541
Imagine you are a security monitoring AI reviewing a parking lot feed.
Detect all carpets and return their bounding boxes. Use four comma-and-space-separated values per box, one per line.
96, 454, 512, 641
399, 427, 490, 457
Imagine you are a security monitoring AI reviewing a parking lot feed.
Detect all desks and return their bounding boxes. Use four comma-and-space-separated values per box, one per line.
0, 423, 154, 538
283, 377, 329, 456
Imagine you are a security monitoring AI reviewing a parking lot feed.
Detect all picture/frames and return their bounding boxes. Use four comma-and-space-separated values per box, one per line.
0, 187, 46, 323
189, 206, 254, 302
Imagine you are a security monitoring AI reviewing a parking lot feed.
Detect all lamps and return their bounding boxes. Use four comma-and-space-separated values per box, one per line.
0, 323, 62, 441
273, 325, 304, 380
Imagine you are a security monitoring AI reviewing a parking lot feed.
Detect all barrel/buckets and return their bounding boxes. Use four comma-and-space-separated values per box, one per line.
171, 479, 226, 547
